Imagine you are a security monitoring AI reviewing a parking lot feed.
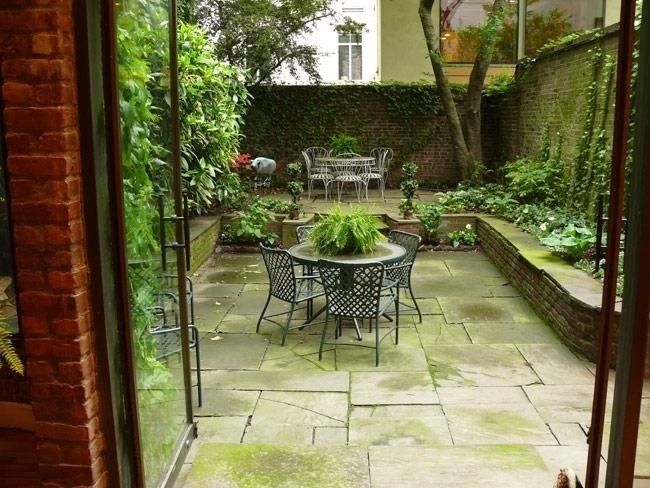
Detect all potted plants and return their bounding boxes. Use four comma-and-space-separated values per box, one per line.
328, 135, 359, 158
288, 181, 302, 219
309, 203, 390, 256
400, 180, 418, 219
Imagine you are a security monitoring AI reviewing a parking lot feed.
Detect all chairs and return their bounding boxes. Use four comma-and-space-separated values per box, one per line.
128, 271, 203, 407
258, 225, 424, 368
300, 146, 395, 203
